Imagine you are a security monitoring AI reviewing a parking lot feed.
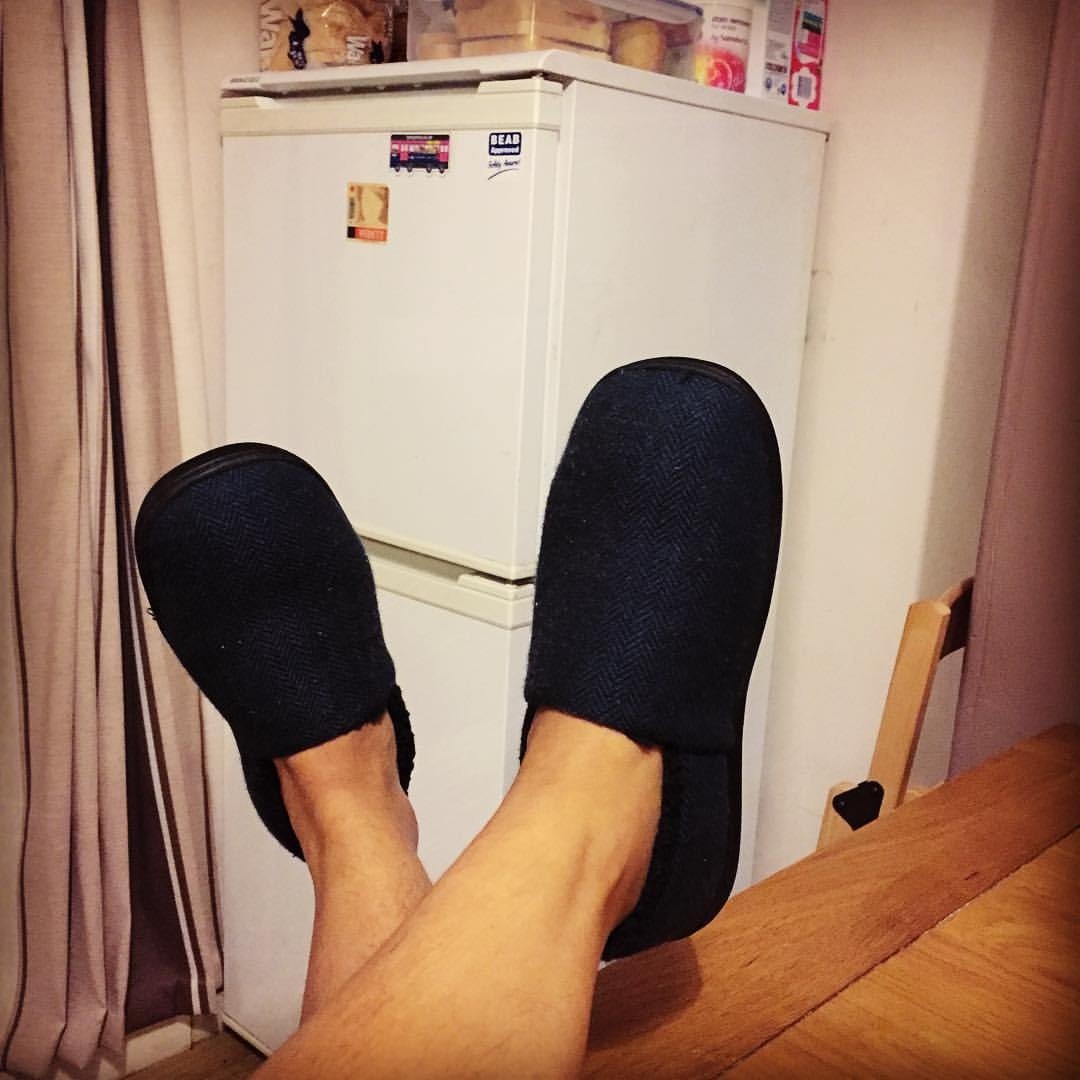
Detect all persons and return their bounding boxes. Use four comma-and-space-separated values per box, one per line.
132, 350, 788, 1080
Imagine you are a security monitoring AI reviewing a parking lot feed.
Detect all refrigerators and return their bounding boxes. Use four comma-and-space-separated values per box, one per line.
203, 49, 824, 1056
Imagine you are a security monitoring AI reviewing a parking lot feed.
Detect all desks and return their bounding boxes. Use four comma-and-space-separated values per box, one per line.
575, 719, 1080, 1080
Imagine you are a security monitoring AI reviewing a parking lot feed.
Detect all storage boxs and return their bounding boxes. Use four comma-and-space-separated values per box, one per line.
745, 0, 828, 111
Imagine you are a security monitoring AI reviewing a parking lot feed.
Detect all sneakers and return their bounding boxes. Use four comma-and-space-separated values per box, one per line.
131, 437, 415, 864
526, 353, 787, 962
